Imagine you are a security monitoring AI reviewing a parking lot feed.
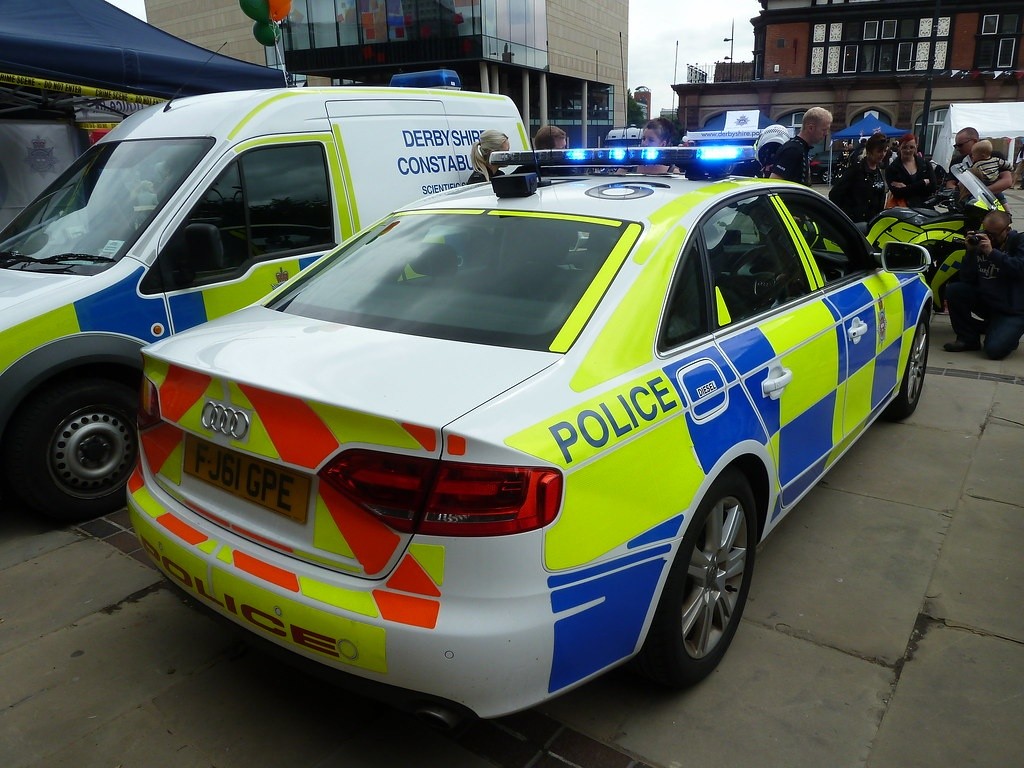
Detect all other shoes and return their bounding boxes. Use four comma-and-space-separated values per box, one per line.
943, 340, 981, 352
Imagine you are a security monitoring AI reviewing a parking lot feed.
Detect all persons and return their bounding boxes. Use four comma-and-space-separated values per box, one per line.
755, 107, 1024, 360
614, 117, 681, 174
465, 129, 512, 185
513, 125, 577, 176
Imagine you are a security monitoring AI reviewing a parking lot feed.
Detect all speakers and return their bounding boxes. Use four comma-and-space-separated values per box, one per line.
491, 173, 538, 199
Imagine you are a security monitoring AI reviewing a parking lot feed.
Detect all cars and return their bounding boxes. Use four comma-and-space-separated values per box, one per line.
809, 151, 842, 183
127, 148, 934, 724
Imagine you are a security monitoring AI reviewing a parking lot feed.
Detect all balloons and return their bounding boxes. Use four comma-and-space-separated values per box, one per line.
241, 0, 292, 47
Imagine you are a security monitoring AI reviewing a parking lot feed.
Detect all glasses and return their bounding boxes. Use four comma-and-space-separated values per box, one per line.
983, 224, 1009, 237
953, 139, 973, 149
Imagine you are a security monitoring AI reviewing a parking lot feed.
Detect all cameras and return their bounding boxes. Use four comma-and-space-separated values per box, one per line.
968, 230, 985, 245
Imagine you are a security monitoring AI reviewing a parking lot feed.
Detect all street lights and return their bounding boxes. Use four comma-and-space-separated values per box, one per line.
724, 38, 733, 81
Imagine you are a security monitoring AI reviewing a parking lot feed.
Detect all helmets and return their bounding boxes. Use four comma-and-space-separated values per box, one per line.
753, 124, 790, 167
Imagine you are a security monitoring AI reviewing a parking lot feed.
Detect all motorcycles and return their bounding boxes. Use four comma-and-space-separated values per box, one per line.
821, 161, 1007, 332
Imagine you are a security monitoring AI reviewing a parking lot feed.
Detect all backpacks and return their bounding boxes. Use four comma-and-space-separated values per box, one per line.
930, 162, 947, 184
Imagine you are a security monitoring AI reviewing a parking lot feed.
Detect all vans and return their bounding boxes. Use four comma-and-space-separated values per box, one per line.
0, 70, 533, 526
605, 126, 644, 148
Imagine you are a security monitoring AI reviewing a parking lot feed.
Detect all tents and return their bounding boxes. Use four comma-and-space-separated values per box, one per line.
0, 0, 292, 234
932, 102, 1024, 173
827, 114, 912, 187
